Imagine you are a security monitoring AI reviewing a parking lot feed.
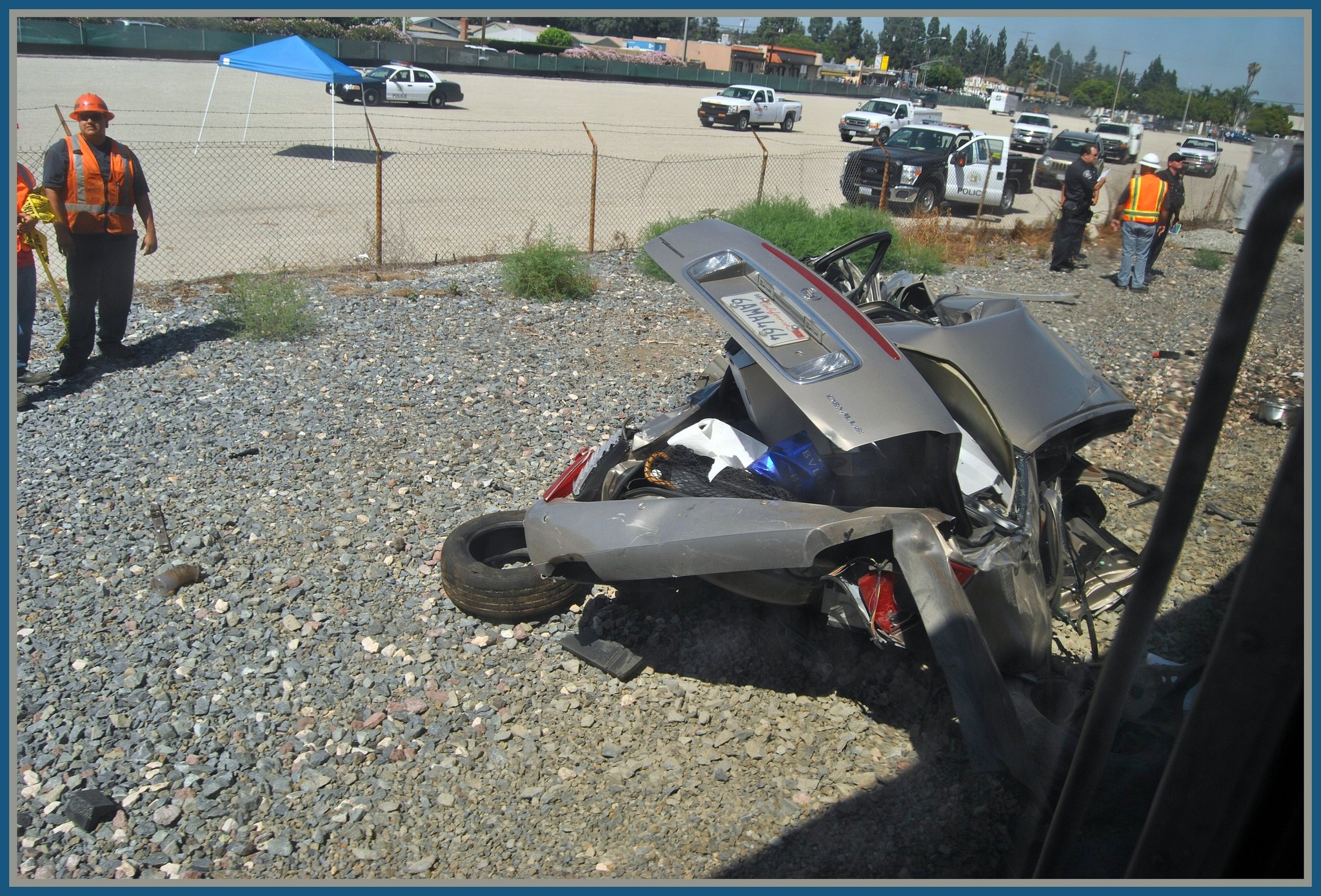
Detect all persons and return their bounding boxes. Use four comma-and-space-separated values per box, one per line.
833, 74, 988, 105
1109, 151, 1171, 294
1049, 142, 1105, 274
1060, 164, 1100, 212
41, 91, 160, 376
15, 124, 57, 414
1224, 130, 1231, 142
1212, 126, 1218, 139
1138, 151, 1186, 282
1220, 129, 1225, 142
1207, 128, 1212, 138
1230, 131, 1235, 143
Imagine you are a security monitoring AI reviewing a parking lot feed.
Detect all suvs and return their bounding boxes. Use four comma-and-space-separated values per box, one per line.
1086, 123, 1141, 163
1175, 138, 1225, 178
1035, 130, 1105, 191
1011, 112, 1056, 154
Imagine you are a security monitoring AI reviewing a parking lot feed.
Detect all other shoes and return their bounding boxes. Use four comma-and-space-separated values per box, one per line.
1050, 262, 1074, 272
1131, 285, 1149, 293
1069, 253, 1086, 259
1117, 283, 1127, 290
1142, 272, 1155, 281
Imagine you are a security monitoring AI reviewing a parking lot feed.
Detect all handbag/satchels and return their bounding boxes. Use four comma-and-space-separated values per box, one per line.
1062, 200, 1084, 216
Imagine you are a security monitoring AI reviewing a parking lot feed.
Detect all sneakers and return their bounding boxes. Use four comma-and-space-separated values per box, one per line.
102, 343, 139, 359
17, 369, 52, 387
17, 390, 28, 408
55, 356, 87, 377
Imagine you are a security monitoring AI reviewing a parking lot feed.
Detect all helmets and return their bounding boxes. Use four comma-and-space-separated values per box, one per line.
1137, 152, 1161, 169
69, 93, 115, 122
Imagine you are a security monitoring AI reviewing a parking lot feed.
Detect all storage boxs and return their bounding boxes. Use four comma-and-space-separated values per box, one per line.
616, 444, 800, 503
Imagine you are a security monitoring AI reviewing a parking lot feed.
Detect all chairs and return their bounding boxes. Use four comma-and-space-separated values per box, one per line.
917, 135, 939, 153
1207, 144, 1215, 150
737, 90, 748, 100
753, 92, 764, 102
1076, 144, 1083, 153
879, 106, 886, 113
768, 96, 773, 102
1062, 140, 1072, 149
1038, 119, 1048, 126
1186, 142, 1194, 148
1022, 117, 1030, 123
897, 108, 905, 116
955, 139, 970, 154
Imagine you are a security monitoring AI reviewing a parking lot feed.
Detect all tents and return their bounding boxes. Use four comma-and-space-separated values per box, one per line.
192, 32, 374, 169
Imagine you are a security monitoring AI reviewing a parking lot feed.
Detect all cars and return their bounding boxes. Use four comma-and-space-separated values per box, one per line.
1091, 113, 1113, 123
326, 60, 463, 109
1224, 130, 1255, 145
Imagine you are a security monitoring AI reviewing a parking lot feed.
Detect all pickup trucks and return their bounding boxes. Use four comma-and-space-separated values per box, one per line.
699, 84, 804, 133
837, 96, 942, 147
840, 118, 1034, 219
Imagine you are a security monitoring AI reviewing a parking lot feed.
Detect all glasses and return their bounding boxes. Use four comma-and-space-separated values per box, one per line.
1175, 158, 1185, 162
78, 113, 106, 122
1089, 153, 1098, 157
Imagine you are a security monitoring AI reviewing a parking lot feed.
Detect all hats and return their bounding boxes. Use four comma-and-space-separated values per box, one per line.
1168, 153, 1186, 161
